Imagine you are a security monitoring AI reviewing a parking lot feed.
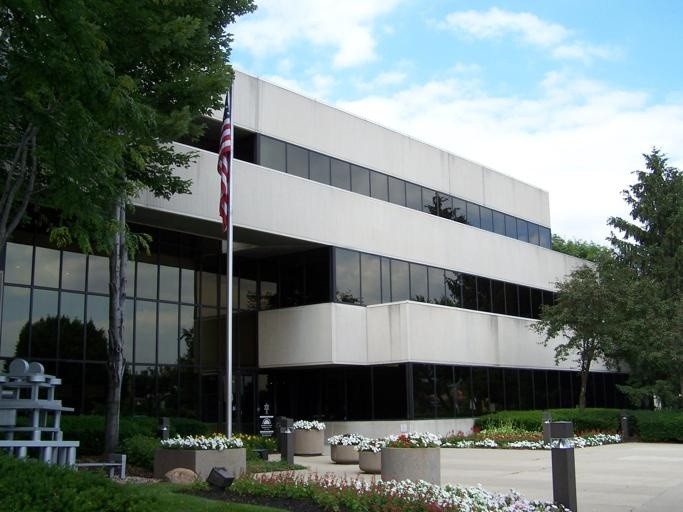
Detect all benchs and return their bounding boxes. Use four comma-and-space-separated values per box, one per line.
75, 453, 127, 481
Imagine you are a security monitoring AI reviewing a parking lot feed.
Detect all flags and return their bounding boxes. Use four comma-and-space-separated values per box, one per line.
217, 90, 231, 234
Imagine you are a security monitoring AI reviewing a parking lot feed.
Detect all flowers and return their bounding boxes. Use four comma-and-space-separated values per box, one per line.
160, 432, 245, 451
326, 432, 365, 446
381, 431, 442, 449
292, 419, 326, 431
353, 438, 385, 454
